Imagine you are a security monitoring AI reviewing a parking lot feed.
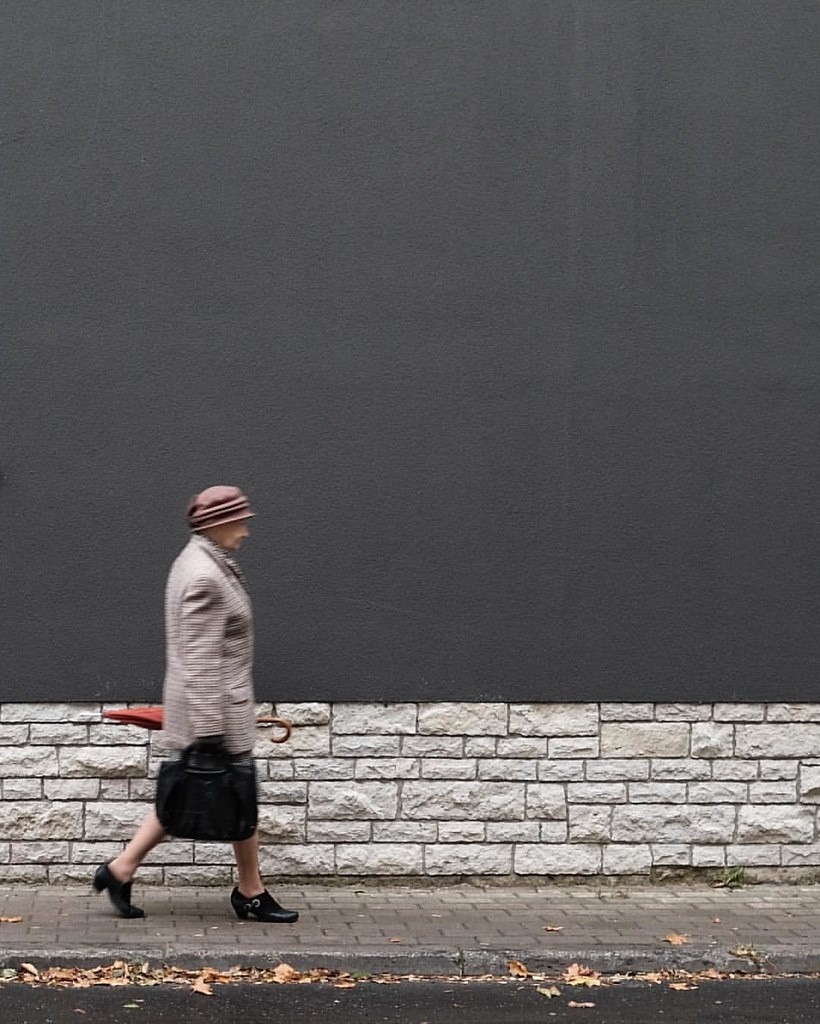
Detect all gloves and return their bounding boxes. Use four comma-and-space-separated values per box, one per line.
197, 735, 223, 759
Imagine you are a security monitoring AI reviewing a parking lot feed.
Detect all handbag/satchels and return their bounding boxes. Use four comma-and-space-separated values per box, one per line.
154, 741, 258, 842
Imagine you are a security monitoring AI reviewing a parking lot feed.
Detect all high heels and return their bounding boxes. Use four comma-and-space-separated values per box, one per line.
230, 886, 298, 923
94, 862, 144, 917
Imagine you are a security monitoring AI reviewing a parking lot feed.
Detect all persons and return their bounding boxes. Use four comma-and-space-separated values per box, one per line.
91, 483, 301, 925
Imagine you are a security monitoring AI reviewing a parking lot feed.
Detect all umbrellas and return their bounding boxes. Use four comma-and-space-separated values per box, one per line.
79, 706, 293, 744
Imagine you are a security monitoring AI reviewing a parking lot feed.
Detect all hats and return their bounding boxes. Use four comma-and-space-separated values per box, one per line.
186, 485, 257, 531
